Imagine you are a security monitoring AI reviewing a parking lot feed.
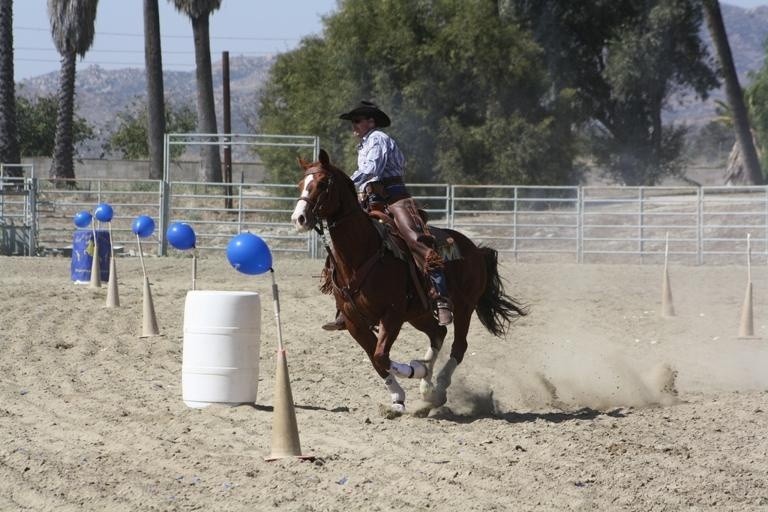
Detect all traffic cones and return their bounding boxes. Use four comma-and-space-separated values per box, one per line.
265, 352, 314, 461
106, 259, 119, 307
140, 278, 163, 337
90, 245, 101, 288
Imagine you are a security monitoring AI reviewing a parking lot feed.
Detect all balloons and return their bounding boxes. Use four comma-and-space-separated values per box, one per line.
166, 220, 197, 251
72, 210, 93, 228
225, 231, 273, 276
95, 202, 114, 222
131, 214, 154, 240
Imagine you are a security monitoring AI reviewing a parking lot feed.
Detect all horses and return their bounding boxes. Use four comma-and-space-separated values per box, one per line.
290, 147, 531, 423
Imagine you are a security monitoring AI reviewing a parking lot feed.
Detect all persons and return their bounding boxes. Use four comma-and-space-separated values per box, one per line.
319, 99, 454, 332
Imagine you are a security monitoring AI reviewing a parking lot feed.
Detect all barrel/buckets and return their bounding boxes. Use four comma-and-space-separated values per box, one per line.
182, 289, 263, 408
71, 229, 114, 284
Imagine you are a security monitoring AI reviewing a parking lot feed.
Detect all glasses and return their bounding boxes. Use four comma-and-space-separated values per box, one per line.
350, 118, 363, 124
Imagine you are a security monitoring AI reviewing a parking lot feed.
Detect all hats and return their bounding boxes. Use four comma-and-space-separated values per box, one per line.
339, 100, 391, 127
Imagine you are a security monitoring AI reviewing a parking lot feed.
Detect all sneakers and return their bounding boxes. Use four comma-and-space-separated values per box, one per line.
438, 305, 452, 326
322, 311, 346, 330
